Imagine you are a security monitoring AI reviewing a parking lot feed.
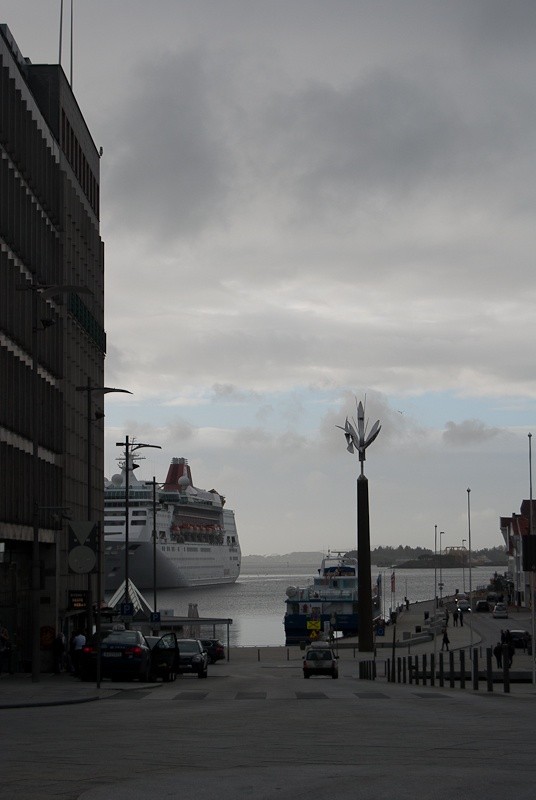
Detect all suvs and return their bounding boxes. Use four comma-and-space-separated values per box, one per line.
303, 642, 339, 678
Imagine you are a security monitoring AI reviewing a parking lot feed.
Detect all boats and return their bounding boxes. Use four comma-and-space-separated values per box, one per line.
284, 550, 383, 646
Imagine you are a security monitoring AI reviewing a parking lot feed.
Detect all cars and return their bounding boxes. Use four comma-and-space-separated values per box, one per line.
508, 629, 531, 651
142, 635, 179, 681
454, 591, 504, 615
198, 638, 225, 664
175, 638, 209, 678
83, 628, 151, 684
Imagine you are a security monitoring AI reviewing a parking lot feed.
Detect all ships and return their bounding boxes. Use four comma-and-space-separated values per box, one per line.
103, 433, 243, 590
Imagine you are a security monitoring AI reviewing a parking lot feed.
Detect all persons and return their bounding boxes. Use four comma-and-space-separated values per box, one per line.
506, 642, 516, 669
494, 642, 503, 669
499, 630, 506, 643
73, 630, 86, 665
439, 627, 450, 652
504, 629, 513, 645
328, 627, 335, 643
452, 610, 458, 627
460, 611, 464, 627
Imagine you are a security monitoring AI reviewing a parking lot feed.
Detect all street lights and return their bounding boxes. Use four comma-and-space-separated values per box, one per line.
76, 378, 134, 692
140, 474, 172, 624
113, 434, 163, 626
